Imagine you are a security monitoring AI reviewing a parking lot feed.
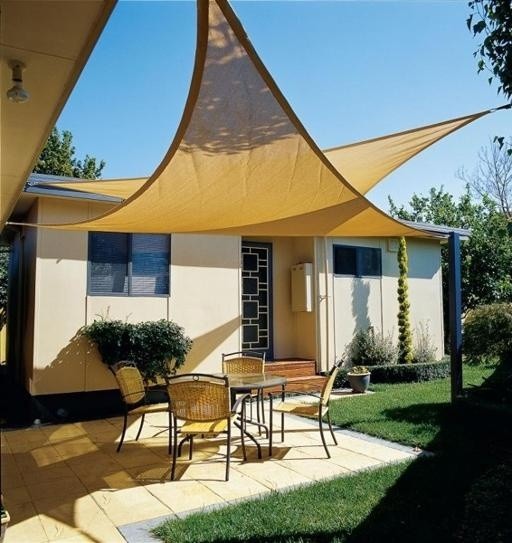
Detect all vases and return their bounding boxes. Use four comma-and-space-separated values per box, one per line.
347, 372, 370, 392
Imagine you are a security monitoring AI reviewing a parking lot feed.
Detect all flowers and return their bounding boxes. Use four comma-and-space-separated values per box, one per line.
345, 364, 372, 374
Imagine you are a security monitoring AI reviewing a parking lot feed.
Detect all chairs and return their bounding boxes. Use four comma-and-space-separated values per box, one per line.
108, 351, 344, 482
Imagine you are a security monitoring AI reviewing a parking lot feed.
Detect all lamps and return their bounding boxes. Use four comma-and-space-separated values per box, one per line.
7, 58, 31, 104
